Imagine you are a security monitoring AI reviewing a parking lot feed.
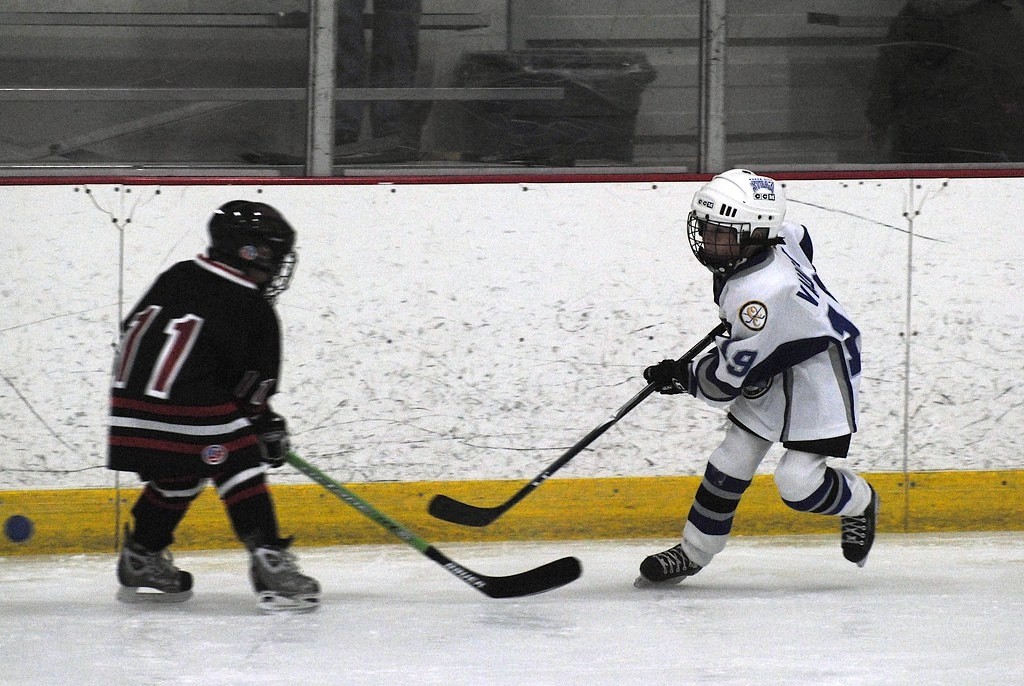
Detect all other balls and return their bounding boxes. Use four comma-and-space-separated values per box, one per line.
4, 512, 33, 543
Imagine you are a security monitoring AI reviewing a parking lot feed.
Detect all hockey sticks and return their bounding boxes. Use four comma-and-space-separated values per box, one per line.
282, 445, 591, 608
422, 317, 733, 530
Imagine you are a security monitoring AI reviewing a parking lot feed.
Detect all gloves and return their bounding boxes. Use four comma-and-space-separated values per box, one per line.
258, 413, 290, 468
643, 359, 697, 398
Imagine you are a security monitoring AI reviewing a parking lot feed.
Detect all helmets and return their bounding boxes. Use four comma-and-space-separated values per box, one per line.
209, 200, 298, 299
686, 168, 785, 275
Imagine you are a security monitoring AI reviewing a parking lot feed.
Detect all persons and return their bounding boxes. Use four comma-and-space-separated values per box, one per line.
334, 0, 424, 163
107, 200, 321, 610
634, 169, 877, 588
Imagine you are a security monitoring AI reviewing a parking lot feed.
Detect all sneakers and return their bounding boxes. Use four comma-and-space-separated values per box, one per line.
839, 481, 878, 568
242, 538, 320, 612
631, 544, 703, 589
116, 521, 192, 602
